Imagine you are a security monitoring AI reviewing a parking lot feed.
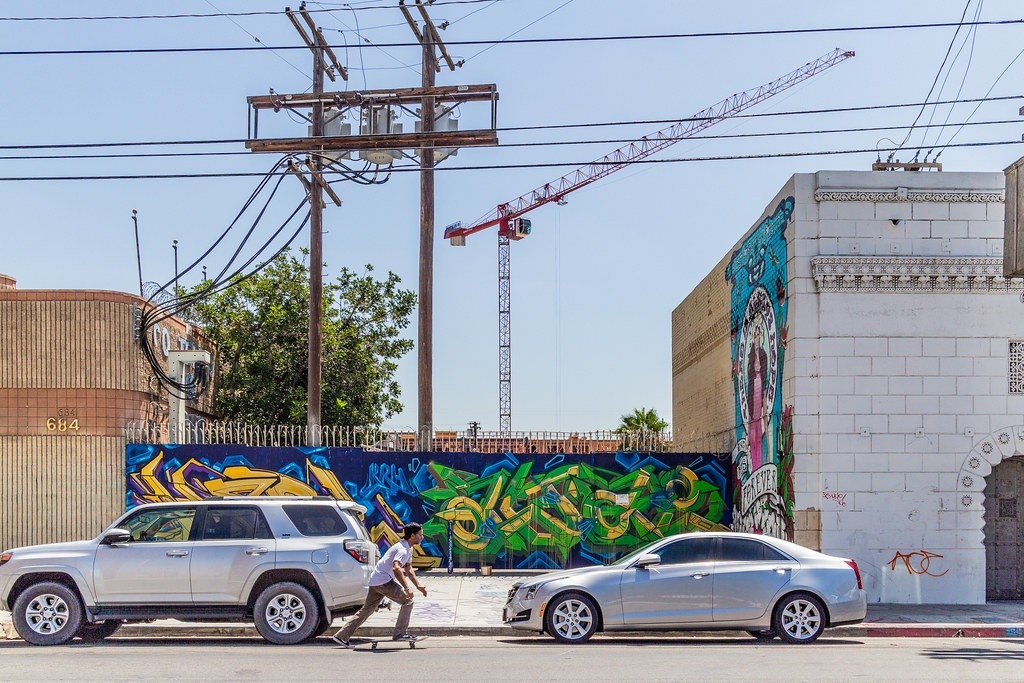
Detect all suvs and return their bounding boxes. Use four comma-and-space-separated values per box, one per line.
0, 494, 383, 647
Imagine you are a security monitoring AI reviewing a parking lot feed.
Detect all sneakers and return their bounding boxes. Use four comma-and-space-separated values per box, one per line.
398, 634, 416, 641
333, 636, 356, 648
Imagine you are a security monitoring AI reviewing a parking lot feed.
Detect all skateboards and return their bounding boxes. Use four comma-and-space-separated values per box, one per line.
358, 635, 429, 650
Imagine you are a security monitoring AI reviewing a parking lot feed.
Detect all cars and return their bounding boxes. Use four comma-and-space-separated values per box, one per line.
502, 532, 868, 643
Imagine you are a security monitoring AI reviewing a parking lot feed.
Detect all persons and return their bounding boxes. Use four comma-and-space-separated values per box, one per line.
333, 522, 427, 648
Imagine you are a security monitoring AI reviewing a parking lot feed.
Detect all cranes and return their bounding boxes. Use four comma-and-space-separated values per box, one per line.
442, 45, 857, 438
